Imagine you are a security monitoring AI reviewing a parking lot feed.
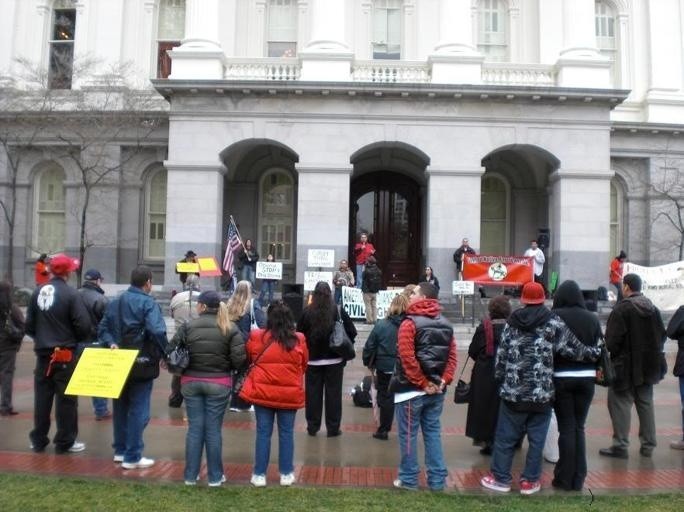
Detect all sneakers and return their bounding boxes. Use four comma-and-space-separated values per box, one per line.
185, 473, 226, 486
98, 410, 112, 421
251, 472, 267, 488
671, 439, 684, 450
481, 475, 512, 492
67, 441, 84, 452
393, 480, 418, 490
1, 410, 19, 414
480, 446, 493, 454
114, 455, 154, 469
280, 473, 295, 486
230, 405, 255, 412
519, 478, 541, 495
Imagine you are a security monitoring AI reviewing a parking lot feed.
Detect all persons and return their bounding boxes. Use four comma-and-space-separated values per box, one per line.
548, 280, 606, 491
25, 254, 111, 453
352, 232, 375, 286
454, 238, 475, 280
163, 290, 248, 487
332, 260, 354, 304
388, 284, 456, 494
362, 285, 417, 441
598, 274, 668, 459
296, 282, 357, 438
236, 300, 309, 488
98, 266, 172, 470
166, 253, 277, 410
666, 305, 684, 450
466, 295, 512, 453
611, 250, 627, 303
524, 239, 545, 284
418, 266, 440, 290
479, 283, 602, 496
0, 281, 26, 415
361, 256, 382, 324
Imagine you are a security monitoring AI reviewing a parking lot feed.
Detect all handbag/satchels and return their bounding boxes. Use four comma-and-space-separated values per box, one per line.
350, 375, 373, 407
164, 342, 191, 375
329, 319, 355, 360
121, 325, 159, 380
250, 298, 260, 329
594, 343, 617, 386
455, 380, 471, 403
4, 316, 23, 343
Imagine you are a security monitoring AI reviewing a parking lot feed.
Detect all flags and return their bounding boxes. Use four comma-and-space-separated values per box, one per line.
223, 218, 242, 277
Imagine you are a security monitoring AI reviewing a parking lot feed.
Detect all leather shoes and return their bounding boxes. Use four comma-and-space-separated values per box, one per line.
373, 431, 387, 439
598, 448, 627, 458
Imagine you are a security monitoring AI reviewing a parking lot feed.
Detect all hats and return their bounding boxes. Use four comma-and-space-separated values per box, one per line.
84, 269, 105, 281
520, 282, 546, 305
190, 290, 221, 308
50, 254, 82, 274
184, 251, 196, 257
